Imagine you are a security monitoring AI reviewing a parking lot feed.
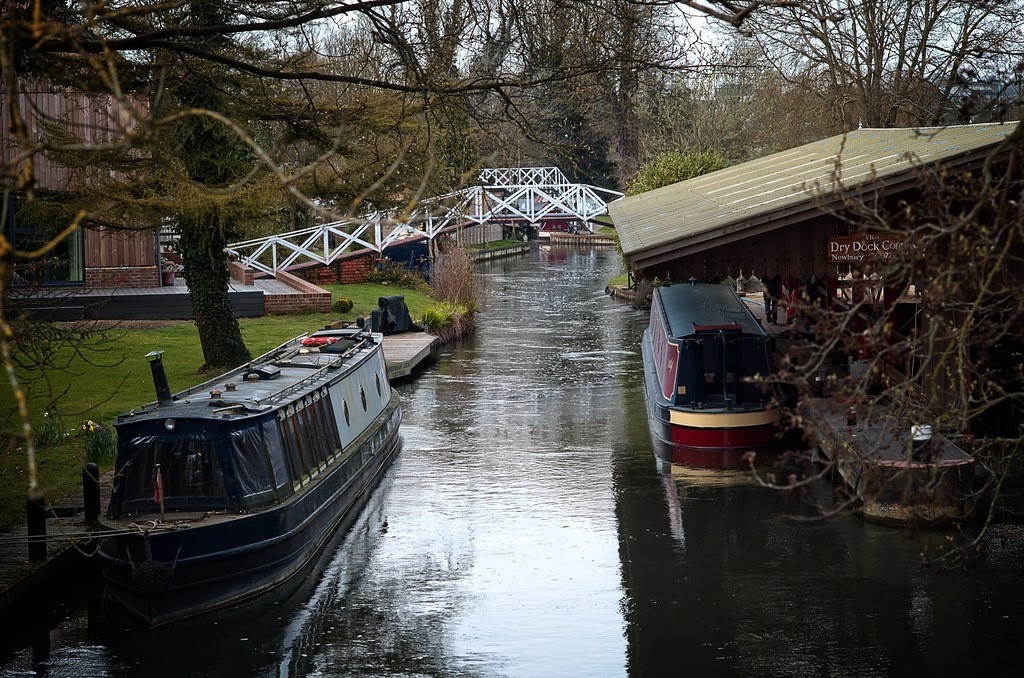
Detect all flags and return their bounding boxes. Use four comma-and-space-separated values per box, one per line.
153, 473, 163, 503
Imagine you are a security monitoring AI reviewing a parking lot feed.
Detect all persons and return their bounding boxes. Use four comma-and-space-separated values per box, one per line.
762, 275, 779, 324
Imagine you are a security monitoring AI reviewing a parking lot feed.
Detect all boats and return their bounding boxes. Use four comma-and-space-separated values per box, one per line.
76, 326, 404, 645
516, 192, 573, 239
639, 279, 789, 487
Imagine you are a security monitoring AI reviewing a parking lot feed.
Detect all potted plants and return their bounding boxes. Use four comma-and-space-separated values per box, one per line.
162, 265, 176, 286
